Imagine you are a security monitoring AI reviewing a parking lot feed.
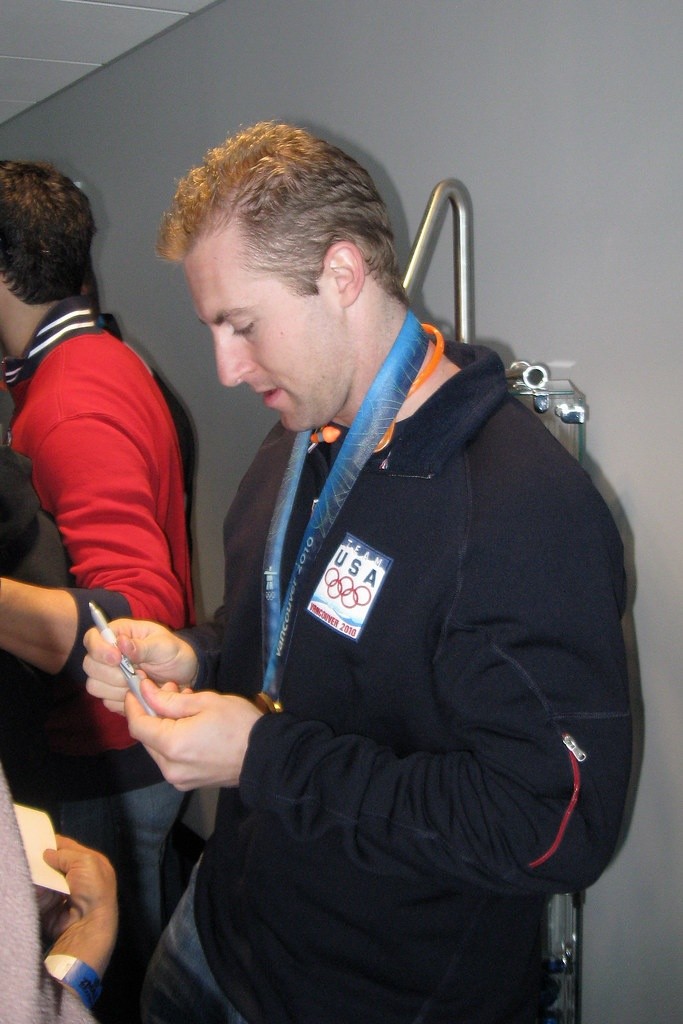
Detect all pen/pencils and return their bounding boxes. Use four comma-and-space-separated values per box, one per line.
83, 600, 159, 719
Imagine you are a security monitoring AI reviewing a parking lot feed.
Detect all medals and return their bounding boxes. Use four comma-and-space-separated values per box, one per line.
252, 691, 282, 716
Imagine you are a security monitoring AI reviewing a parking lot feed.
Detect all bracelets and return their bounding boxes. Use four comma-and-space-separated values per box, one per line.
44, 955, 102, 1009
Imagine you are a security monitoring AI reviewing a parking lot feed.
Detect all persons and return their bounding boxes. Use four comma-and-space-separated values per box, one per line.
83, 122, 631, 1024
0, 159, 194, 1024
